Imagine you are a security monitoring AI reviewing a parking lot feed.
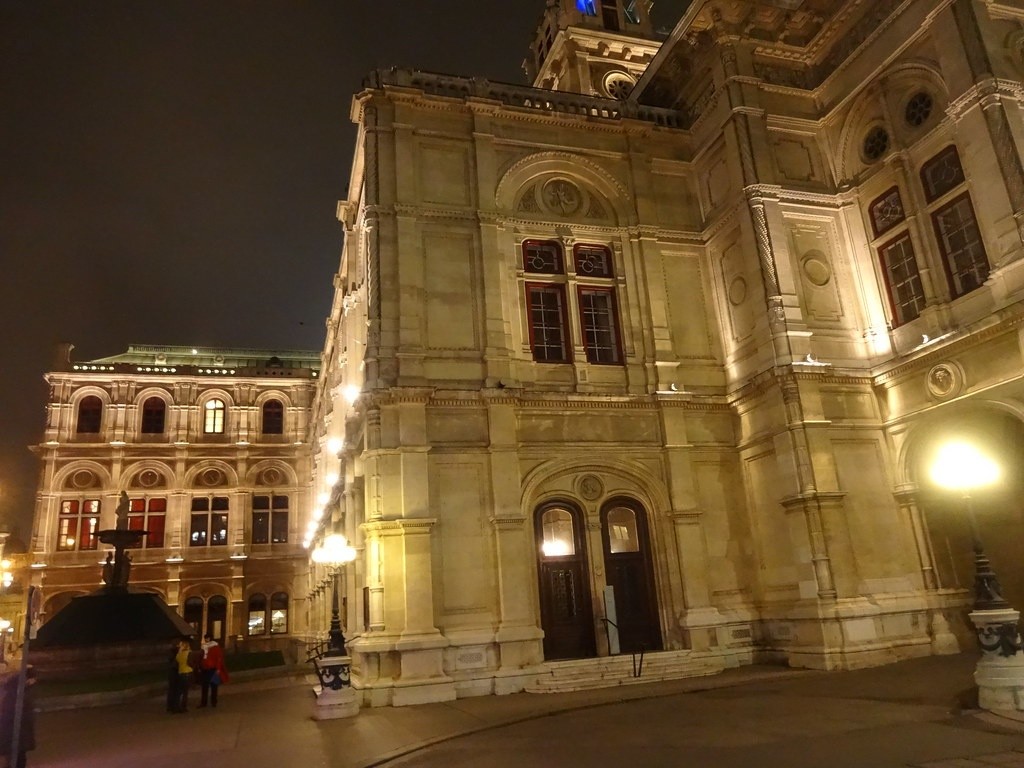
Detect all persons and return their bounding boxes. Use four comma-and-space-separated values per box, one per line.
0, 675, 35, 768
102, 552, 113, 583
115, 492, 129, 530
122, 552, 133, 584
167, 639, 195, 712
199, 633, 224, 708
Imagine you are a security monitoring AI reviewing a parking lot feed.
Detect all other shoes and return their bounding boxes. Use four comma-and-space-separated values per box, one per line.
172, 709, 182, 714
212, 702, 216, 707
197, 703, 207, 708
182, 708, 189, 712
